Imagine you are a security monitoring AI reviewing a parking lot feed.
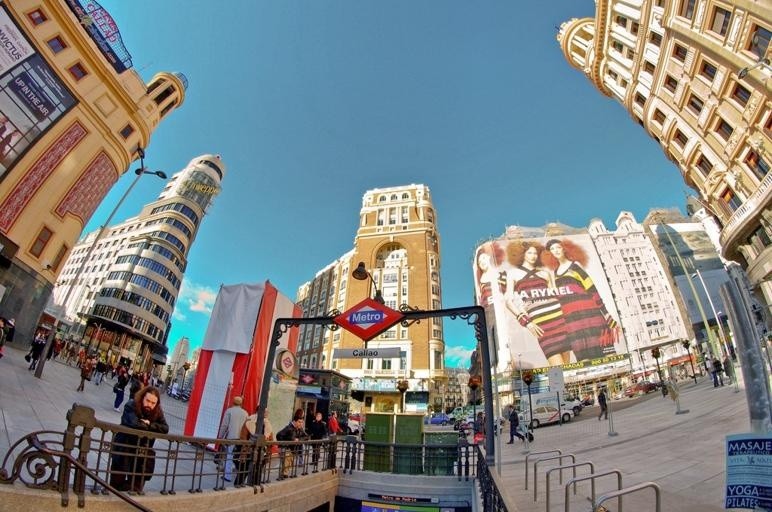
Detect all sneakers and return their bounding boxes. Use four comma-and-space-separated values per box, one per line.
114, 407, 121, 413
522, 436, 524, 442
506, 440, 514, 444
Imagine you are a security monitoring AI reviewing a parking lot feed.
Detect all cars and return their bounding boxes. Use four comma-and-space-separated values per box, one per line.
624, 380, 662, 397
515, 405, 575, 430
423, 412, 456, 425
348, 412, 365, 435
166, 384, 193, 403
461, 412, 507, 436
614, 391, 626, 400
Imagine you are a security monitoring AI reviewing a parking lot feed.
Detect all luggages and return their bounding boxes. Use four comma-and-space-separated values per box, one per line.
526, 432, 534, 443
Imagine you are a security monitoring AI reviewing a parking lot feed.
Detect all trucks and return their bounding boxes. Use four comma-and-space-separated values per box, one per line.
449, 402, 486, 422
515, 390, 583, 416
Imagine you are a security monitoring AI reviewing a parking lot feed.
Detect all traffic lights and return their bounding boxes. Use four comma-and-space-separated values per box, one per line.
652, 348, 661, 360
682, 341, 688, 350
688, 340, 692, 347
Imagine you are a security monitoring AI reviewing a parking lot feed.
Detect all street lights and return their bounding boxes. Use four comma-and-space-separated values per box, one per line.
91, 319, 108, 360
354, 260, 388, 306
689, 268, 739, 384
69, 282, 99, 343
33, 146, 169, 381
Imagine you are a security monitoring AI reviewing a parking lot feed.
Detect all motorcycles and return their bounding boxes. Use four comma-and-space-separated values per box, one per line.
581, 399, 596, 408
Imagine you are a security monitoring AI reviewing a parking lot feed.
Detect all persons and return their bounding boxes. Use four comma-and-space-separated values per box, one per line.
214, 395, 344, 487
705, 356, 735, 389
474, 412, 486, 449
506, 404, 525, 444
0, 316, 151, 413
597, 388, 608, 421
476, 238, 623, 366
109, 386, 169, 492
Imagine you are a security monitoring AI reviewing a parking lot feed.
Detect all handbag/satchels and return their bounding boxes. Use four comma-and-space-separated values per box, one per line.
25, 355, 31, 362
113, 383, 123, 393
